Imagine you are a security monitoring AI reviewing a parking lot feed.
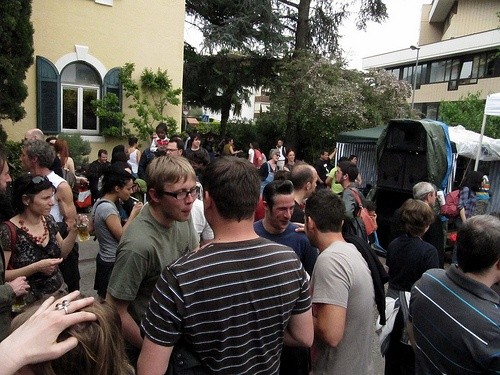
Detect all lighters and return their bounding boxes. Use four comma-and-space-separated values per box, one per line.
139, 202, 141, 204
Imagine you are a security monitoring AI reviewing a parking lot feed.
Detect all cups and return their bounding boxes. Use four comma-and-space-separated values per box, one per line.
436, 190, 446, 206
75, 224, 91, 242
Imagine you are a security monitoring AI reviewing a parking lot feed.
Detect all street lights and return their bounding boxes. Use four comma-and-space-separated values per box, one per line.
410, 45, 421, 113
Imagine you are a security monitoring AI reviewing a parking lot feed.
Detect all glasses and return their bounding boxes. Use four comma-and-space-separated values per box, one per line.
28, 174, 49, 187
158, 186, 199, 200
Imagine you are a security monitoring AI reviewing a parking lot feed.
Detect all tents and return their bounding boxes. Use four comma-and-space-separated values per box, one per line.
335, 117, 500, 214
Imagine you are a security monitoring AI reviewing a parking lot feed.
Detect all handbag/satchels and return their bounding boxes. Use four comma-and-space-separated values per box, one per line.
134, 178, 147, 193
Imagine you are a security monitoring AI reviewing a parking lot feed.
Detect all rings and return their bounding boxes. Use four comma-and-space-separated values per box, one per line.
55, 300, 70, 314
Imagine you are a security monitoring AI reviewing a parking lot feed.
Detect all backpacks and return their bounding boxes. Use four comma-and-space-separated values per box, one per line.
441, 189, 460, 218
349, 188, 378, 237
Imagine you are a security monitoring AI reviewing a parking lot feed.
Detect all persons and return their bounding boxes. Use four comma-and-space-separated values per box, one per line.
0, 123, 500, 375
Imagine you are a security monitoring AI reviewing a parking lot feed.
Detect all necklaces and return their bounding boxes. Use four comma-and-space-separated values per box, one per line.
17, 213, 48, 244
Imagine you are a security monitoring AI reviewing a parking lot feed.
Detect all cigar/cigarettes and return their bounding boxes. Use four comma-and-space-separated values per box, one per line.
129, 196, 139, 201
